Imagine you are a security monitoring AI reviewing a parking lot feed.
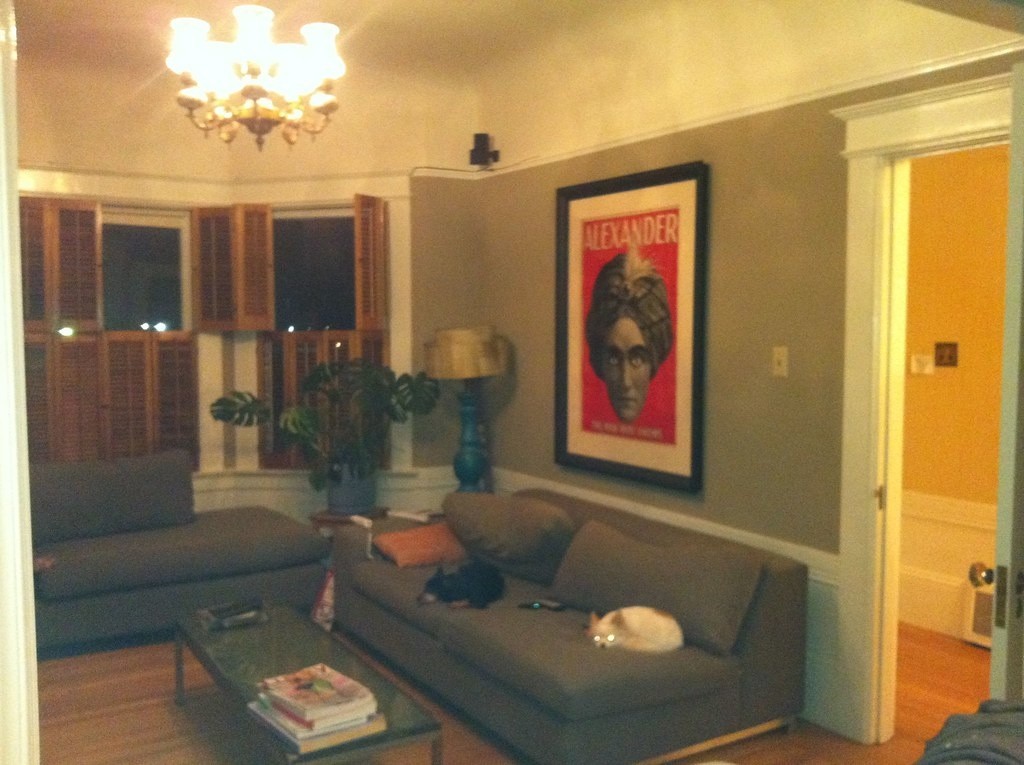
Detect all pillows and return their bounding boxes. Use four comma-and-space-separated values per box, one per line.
374, 522, 470, 569
552, 522, 764, 657
443, 492, 576, 580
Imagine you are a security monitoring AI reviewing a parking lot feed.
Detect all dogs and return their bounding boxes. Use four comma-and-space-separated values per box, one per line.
416, 567, 507, 609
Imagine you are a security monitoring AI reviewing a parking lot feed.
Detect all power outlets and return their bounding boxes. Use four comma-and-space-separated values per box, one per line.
934, 343, 957, 367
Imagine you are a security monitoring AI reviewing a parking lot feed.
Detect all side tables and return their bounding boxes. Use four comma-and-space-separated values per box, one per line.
306, 505, 389, 533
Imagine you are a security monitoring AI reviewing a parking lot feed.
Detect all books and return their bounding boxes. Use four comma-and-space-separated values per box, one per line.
244, 662, 388, 755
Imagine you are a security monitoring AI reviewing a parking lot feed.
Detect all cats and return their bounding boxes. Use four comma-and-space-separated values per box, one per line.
588, 606, 684, 652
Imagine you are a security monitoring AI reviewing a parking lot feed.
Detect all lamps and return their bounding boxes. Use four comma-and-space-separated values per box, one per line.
166, 2, 346, 150
424, 327, 515, 495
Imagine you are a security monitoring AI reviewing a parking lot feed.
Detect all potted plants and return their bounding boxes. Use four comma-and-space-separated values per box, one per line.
208, 355, 446, 515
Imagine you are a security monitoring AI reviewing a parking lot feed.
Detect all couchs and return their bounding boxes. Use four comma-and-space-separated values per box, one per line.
31, 449, 337, 663
323, 485, 808, 765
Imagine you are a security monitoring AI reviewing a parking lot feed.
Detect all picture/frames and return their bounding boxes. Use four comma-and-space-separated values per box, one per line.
553, 158, 712, 490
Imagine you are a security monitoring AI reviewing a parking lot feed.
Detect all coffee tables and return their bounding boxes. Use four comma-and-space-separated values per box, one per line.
168, 605, 443, 765
